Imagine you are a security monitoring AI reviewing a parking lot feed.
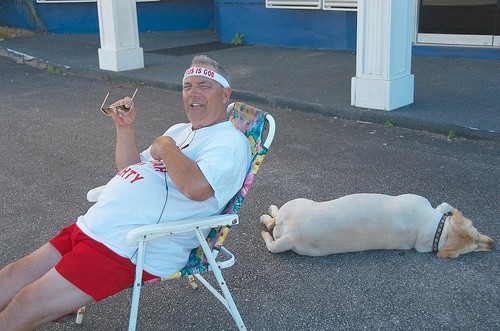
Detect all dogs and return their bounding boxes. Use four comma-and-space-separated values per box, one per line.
260, 192, 495, 258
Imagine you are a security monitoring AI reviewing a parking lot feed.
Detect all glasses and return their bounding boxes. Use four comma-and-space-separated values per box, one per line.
100, 83, 145, 116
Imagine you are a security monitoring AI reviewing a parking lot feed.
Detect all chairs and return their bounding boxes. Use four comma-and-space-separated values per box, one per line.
75, 101, 276, 331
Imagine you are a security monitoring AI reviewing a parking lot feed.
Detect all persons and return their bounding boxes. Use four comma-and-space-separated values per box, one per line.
0, 55, 252, 331
